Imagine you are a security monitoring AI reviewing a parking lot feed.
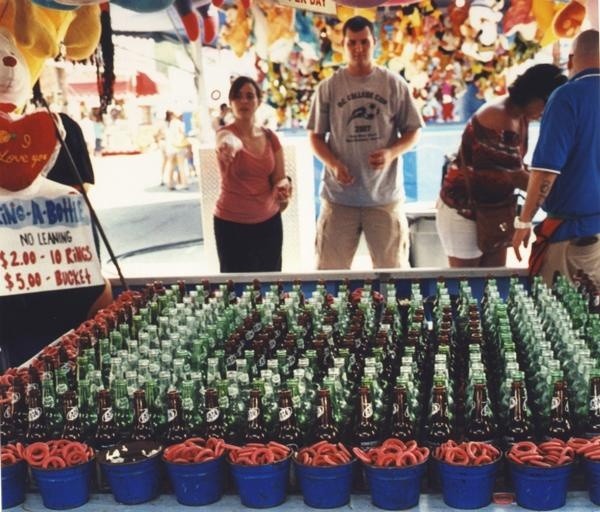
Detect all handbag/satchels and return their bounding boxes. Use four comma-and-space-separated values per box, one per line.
460, 90, 525, 251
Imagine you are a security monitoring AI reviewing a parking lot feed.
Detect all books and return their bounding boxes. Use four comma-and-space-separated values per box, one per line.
373, 3, 511, 123
266, 75, 323, 128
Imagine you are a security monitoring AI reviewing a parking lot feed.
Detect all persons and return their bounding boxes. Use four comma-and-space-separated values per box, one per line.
160, 110, 190, 190
30, 84, 100, 271
307, 17, 425, 269
213, 77, 292, 271
435, 64, 568, 268
513, 30, 599, 284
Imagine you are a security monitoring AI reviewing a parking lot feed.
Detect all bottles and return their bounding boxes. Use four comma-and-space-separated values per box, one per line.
427, 387, 453, 486
202, 390, 228, 445
250, 378, 272, 423
353, 388, 383, 490
1, 400, 16, 448
359, 376, 383, 433
586, 376, 600, 433
93, 393, 122, 493
542, 382, 575, 451
131, 391, 157, 444
61, 395, 87, 448
112, 381, 133, 437
164, 391, 190, 451
285, 380, 306, 427
309, 391, 340, 447
226, 384, 245, 438
0, 269, 599, 407
245, 392, 269, 445
389, 386, 417, 443
24, 392, 52, 491
180, 380, 203, 437
466, 386, 498, 449
274, 390, 304, 491
502, 382, 536, 484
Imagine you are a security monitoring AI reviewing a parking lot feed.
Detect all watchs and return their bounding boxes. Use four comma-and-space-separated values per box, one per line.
513, 218, 534, 230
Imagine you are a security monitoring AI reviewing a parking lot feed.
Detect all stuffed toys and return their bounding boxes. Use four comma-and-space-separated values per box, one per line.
0, 27, 78, 205
11, 1, 111, 85
512, 1, 587, 60
